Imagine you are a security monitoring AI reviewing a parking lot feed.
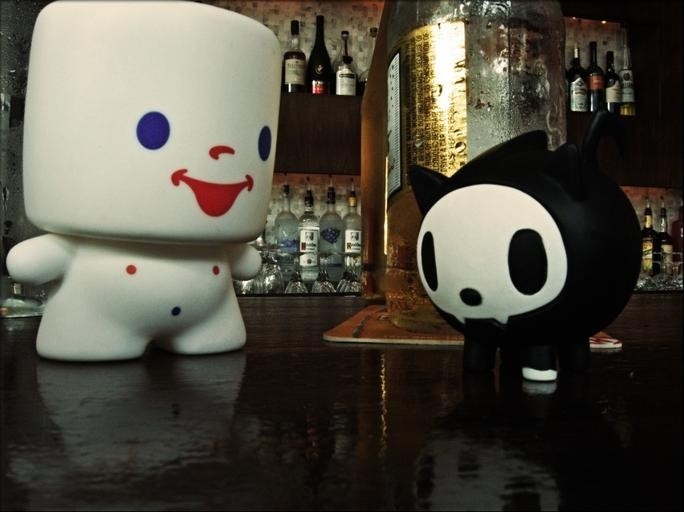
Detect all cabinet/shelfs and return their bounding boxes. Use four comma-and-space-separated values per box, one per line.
194, 0, 684, 348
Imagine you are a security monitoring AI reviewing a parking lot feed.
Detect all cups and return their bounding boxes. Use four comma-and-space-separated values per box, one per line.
633, 251, 683, 291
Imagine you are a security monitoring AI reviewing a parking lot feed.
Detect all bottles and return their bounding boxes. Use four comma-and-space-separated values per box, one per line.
274, 172, 298, 260
566, 41, 588, 114
297, 177, 319, 266
332, 30, 359, 96
284, 19, 307, 92
604, 50, 620, 115
587, 40, 604, 113
306, 14, 332, 94
617, 42, 636, 115
232, 252, 364, 295
343, 177, 363, 266
318, 174, 343, 265
636, 195, 684, 277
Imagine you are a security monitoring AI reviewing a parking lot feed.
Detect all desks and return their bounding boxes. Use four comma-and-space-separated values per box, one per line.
0, 295, 682, 511
